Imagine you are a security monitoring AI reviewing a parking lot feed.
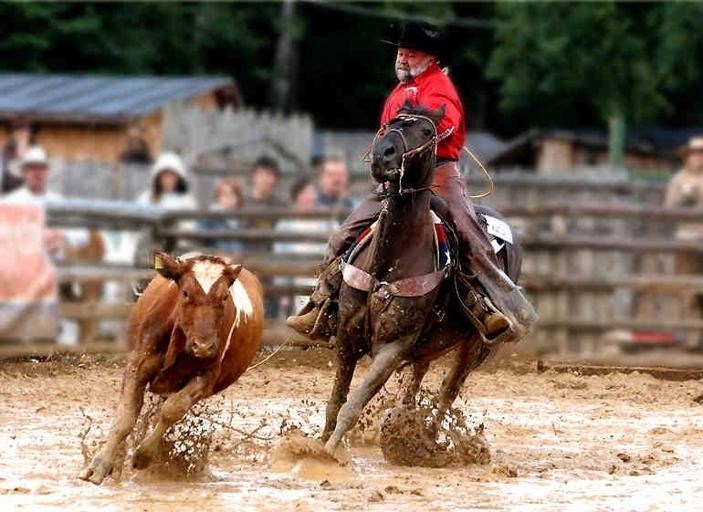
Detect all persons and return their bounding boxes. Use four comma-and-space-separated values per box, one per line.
665, 136, 703, 351
286, 21, 510, 337
0, 126, 360, 317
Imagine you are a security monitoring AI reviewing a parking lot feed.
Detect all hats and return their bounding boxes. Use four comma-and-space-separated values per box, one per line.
382, 19, 444, 54
678, 135, 702, 157
8, 146, 61, 180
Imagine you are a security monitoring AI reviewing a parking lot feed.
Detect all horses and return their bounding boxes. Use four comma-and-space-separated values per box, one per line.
320, 98, 524, 458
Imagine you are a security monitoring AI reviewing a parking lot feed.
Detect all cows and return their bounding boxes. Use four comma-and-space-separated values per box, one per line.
76, 248, 265, 486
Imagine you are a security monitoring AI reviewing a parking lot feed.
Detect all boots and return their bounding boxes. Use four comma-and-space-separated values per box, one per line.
286, 290, 333, 340
463, 288, 510, 340
